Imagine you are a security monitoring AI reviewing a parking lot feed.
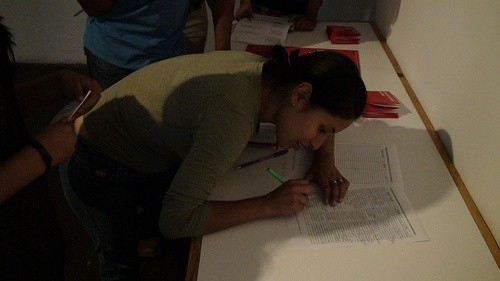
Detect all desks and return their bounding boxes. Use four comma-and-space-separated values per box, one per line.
188, 20, 500, 281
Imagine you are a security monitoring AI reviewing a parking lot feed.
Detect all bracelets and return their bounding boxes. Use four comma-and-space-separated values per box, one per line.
28, 140, 53, 172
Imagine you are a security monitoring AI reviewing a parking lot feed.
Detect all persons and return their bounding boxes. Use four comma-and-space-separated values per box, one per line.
77, 0, 236, 257
234, 0, 322, 32
58, 46, 367, 281
0, 15, 102, 281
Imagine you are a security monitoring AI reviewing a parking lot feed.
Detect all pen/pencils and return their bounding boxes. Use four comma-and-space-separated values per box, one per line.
235, 149, 289, 169
267, 167, 312, 208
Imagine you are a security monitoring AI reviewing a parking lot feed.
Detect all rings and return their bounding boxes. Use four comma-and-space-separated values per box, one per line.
337, 178, 344, 183
330, 178, 338, 186
